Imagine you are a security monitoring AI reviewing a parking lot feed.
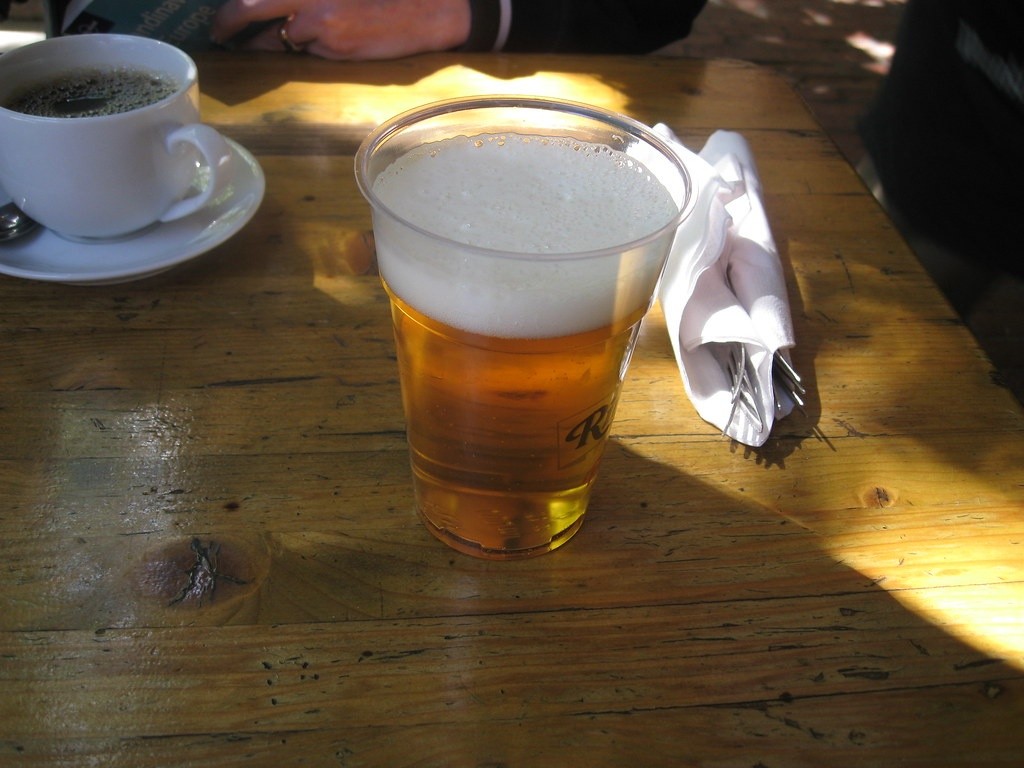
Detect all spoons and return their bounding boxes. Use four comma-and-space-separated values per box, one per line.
0, 202, 39, 241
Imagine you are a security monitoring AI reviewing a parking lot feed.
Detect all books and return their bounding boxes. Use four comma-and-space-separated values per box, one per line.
43, 0, 224, 50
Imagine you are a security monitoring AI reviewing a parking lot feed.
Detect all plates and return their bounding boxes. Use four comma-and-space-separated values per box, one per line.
0, 132, 266, 285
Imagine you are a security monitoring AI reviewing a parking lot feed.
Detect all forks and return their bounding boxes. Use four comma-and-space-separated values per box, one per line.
773, 350, 806, 406
705, 342, 745, 436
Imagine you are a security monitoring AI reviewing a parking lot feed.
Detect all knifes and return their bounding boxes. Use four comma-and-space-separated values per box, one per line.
738, 368, 763, 436
772, 384, 782, 411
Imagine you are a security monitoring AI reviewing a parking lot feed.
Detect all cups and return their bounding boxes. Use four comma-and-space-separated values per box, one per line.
355, 93, 699, 561
0, 34, 231, 238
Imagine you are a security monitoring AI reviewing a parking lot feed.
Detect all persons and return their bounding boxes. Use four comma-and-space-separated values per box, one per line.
862, 0, 1024, 405
209, 1, 709, 61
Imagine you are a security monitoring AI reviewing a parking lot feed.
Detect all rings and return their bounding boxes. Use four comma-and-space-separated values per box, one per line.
279, 15, 305, 52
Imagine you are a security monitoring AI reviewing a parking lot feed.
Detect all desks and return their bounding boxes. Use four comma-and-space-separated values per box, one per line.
0, 49, 1024, 768
